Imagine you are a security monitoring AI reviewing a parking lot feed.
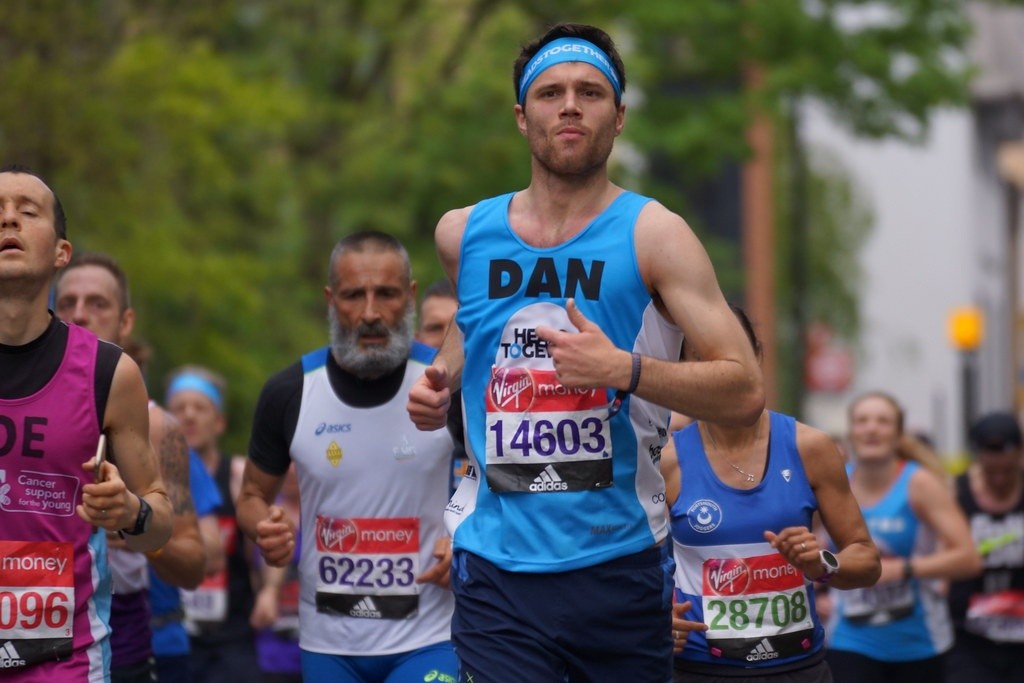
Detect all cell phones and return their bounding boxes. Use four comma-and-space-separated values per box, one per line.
93, 435, 107, 484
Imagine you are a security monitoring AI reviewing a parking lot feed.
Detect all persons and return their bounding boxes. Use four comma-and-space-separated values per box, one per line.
660, 305, 1024, 683
55, 230, 465, 683
407, 20, 767, 683
0, 163, 173, 683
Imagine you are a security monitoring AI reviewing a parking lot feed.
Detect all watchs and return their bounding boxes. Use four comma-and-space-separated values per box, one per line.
117, 497, 153, 540
803, 549, 840, 582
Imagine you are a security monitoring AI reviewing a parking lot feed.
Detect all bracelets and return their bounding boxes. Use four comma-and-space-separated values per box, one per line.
902, 558, 912, 578
604, 353, 642, 421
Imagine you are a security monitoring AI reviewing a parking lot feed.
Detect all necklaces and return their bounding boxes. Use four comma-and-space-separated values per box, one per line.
703, 413, 763, 482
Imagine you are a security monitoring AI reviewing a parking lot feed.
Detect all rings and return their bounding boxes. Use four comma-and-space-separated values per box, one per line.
102, 510, 107, 519
673, 639, 678, 644
676, 631, 680, 639
673, 647, 676, 653
802, 543, 807, 551
